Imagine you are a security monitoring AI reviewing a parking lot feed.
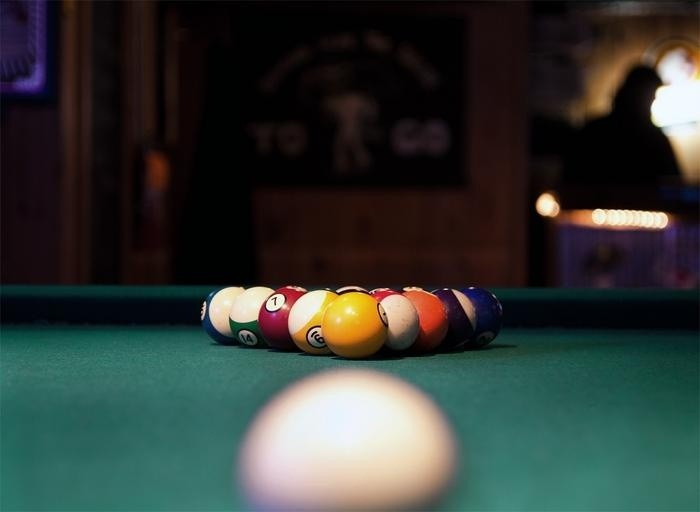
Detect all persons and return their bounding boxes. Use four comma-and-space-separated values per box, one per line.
554, 60, 688, 287
520, 55, 588, 286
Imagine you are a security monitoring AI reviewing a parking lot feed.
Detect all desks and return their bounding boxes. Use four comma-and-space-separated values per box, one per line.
0, 285, 700, 512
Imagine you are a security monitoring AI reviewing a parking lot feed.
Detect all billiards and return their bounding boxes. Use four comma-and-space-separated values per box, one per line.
201, 286, 504, 360
239, 370, 456, 512
